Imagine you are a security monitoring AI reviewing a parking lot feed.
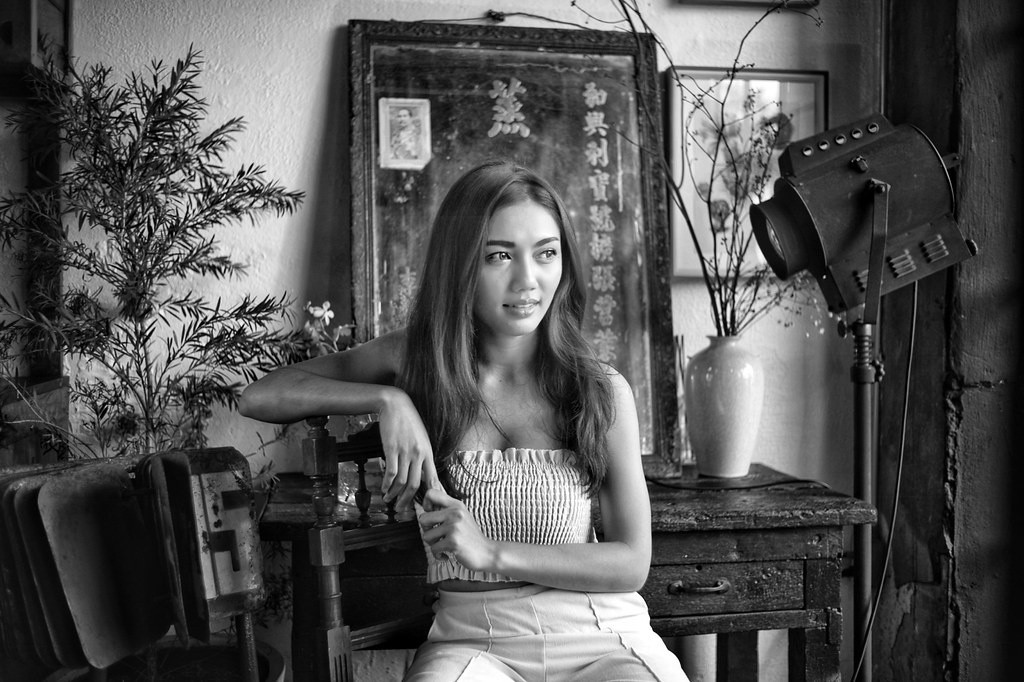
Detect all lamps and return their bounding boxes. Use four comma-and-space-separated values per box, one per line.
747, 113, 979, 682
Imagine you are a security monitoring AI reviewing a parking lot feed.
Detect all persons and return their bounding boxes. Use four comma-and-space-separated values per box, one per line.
389, 108, 421, 160
237, 160, 692, 682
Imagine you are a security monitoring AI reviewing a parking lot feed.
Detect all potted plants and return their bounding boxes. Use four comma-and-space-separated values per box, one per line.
0, 31, 318, 682
488, 0, 843, 478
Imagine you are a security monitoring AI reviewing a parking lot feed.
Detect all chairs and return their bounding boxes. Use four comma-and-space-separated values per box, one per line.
290, 415, 437, 682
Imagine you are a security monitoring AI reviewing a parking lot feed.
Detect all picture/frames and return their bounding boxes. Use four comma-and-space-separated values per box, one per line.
348, 17, 682, 481
667, 65, 831, 279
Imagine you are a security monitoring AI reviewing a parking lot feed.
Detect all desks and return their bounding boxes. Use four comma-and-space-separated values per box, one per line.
250, 462, 877, 682
